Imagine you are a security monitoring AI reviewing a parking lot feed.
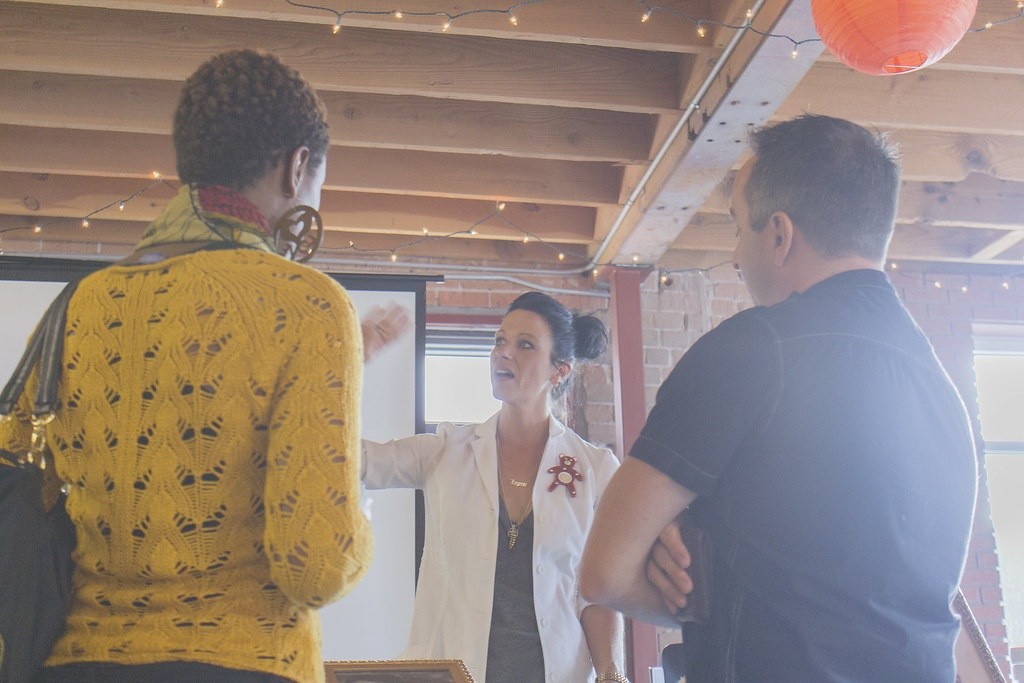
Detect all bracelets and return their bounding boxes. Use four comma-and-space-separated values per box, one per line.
596, 673, 630, 683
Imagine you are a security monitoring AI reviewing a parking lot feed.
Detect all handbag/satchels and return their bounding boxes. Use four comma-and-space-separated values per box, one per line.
0, 275, 86, 683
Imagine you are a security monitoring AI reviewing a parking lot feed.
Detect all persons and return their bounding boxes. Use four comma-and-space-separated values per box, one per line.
579, 115, 977, 683
359, 291, 631, 683
0, 49, 375, 683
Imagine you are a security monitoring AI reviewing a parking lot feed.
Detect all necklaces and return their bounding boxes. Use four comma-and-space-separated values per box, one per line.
496, 433, 541, 487
497, 450, 531, 550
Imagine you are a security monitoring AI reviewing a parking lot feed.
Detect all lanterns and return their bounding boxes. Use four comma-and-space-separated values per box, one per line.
811, 0, 978, 75
